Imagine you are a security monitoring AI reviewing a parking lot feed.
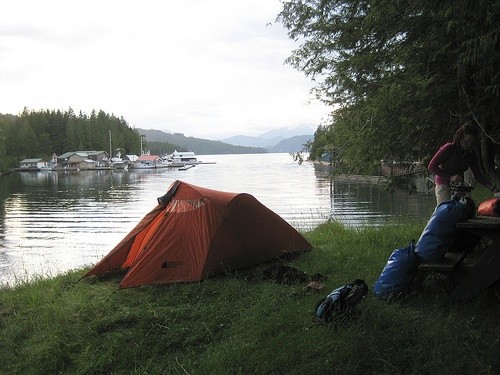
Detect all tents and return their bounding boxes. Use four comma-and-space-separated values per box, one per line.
476, 195, 500, 218
414, 191, 470, 274
366, 236, 423, 309
73, 177, 315, 294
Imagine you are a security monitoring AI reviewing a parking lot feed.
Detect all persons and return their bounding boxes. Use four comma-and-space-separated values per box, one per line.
427, 118, 498, 210
